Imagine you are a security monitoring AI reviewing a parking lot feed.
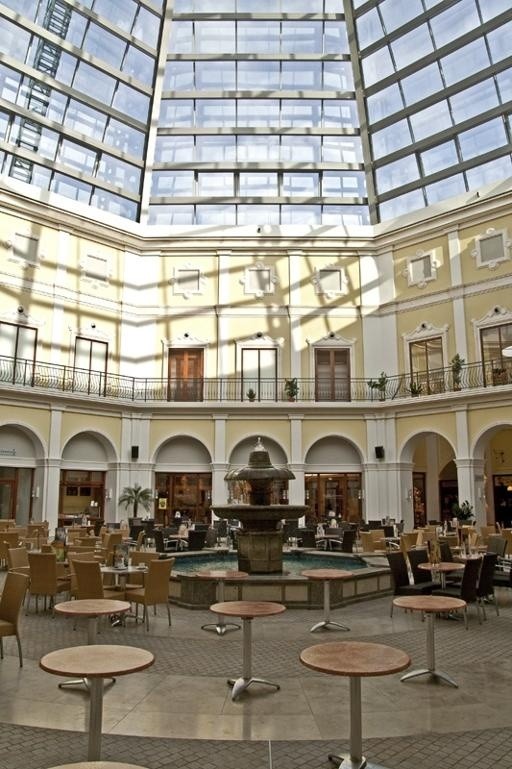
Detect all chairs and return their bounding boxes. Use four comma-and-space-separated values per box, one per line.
384, 516, 510, 620
174, 518, 239, 552
2, 517, 174, 642
282, 514, 405, 553
2, 572, 29, 666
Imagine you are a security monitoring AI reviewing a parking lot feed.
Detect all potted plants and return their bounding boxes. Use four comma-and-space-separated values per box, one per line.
365, 371, 392, 402
401, 381, 424, 398
448, 353, 466, 392
451, 499, 477, 525
117, 481, 158, 525
247, 387, 258, 402
283, 377, 301, 402
489, 366, 509, 385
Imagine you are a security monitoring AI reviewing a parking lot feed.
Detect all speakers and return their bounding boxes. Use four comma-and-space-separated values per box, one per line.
375, 446, 384, 458
131, 445, 139, 458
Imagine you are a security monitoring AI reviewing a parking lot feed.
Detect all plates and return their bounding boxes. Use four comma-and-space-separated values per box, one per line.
136, 566, 147, 569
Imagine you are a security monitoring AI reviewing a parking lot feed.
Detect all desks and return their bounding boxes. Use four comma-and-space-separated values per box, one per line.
300, 568, 357, 634
54, 599, 131, 689
211, 601, 285, 703
393, 596, 467, 690
38, 644, 156, 764
196, 570, 249, 635
299, 641, 412, 769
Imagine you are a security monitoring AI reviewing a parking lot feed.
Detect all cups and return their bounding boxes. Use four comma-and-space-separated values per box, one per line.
139, 562, 147, 567
